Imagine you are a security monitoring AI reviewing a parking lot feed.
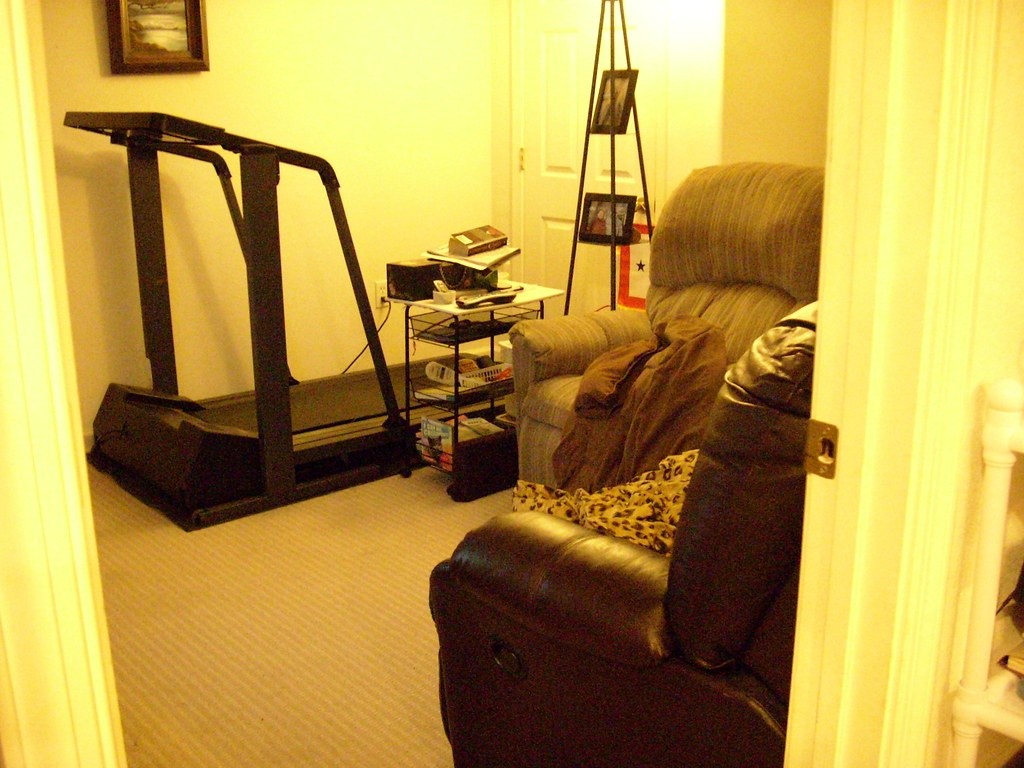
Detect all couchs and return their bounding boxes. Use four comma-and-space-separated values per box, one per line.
429, 301, 817, 768
508, 164, 823, 489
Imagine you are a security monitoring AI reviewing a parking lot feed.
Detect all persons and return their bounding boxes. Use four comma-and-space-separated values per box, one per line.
588, 209, 624, 236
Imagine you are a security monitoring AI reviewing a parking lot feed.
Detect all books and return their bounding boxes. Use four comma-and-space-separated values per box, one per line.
448, 224, 509, 256
416, 413, 507, 467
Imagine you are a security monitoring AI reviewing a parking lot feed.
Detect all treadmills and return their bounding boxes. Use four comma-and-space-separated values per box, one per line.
62, 110, 515, 535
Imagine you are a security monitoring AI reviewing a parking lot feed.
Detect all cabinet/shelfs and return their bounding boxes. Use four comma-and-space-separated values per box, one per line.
386, 281, 563, 482
949, 379, 1024, 768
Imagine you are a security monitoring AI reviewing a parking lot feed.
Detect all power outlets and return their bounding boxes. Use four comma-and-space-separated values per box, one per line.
375, 280, 391, 308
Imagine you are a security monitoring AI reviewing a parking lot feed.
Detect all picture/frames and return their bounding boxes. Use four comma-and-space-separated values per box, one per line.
106, 0, 209, 75
578, 192, 637, 245
591, 70, 639, 134
